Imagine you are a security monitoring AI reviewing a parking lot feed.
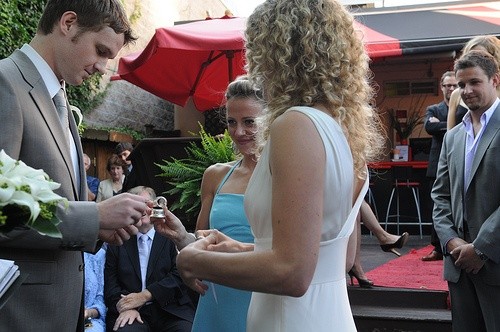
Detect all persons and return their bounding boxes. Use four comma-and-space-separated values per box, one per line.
462, 35, 500, 99
430, 50, 500, 332
0, 0, 153, 332
422, 71, 469, 261
174, 0, 385, 332
153, 73, 269, 332
82, 141, 180, 332
347, 199, 408, 288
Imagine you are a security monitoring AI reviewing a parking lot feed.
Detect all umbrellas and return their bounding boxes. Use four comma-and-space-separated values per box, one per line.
109, 18, 255, 113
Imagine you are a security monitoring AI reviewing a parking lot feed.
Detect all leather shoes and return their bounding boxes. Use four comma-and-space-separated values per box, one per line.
422, 251, 443, 261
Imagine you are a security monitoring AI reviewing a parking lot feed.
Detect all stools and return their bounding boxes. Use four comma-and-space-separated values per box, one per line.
385, 165, 423, 239
365, 172, 379, 237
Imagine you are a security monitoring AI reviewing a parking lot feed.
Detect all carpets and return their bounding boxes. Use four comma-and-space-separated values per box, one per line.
349, 243, 453, 310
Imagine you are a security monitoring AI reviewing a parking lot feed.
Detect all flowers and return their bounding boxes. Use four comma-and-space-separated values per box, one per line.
0, 148, 68, 239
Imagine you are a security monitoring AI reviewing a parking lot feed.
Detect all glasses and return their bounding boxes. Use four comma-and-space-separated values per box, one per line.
442, 83, 457, 88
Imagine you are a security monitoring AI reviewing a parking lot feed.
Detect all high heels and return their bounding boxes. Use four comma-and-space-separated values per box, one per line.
348, 270, 373, 287
380, 232, 408, 257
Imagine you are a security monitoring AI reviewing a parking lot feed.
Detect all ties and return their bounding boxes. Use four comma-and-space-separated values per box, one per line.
53, 88, 70, 150
140, 234, 150, 289
